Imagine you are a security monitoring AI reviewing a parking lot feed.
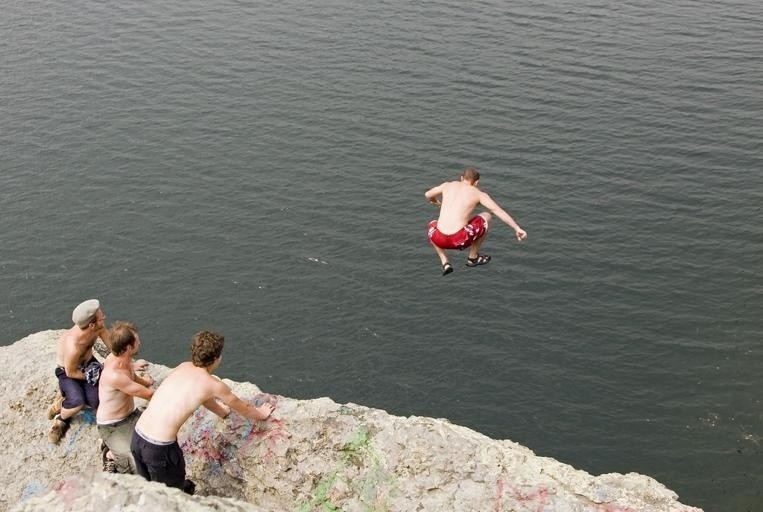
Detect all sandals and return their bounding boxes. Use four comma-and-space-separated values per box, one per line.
465, 253, 491, 267
442, 262, 454, 276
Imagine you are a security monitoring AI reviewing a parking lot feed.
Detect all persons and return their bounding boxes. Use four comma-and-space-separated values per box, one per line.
421, 169, 528, 277
93, 320, 157, 475
43, 297, 115, 445
129, 328, 275, 496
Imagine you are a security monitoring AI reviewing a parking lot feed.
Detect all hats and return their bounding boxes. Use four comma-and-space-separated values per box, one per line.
71, 298, 101, 329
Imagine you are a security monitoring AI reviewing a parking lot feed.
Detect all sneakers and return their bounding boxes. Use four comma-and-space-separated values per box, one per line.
48, 419, 66, 445
45, 395, 64, 419
101, 440, 116, 474
